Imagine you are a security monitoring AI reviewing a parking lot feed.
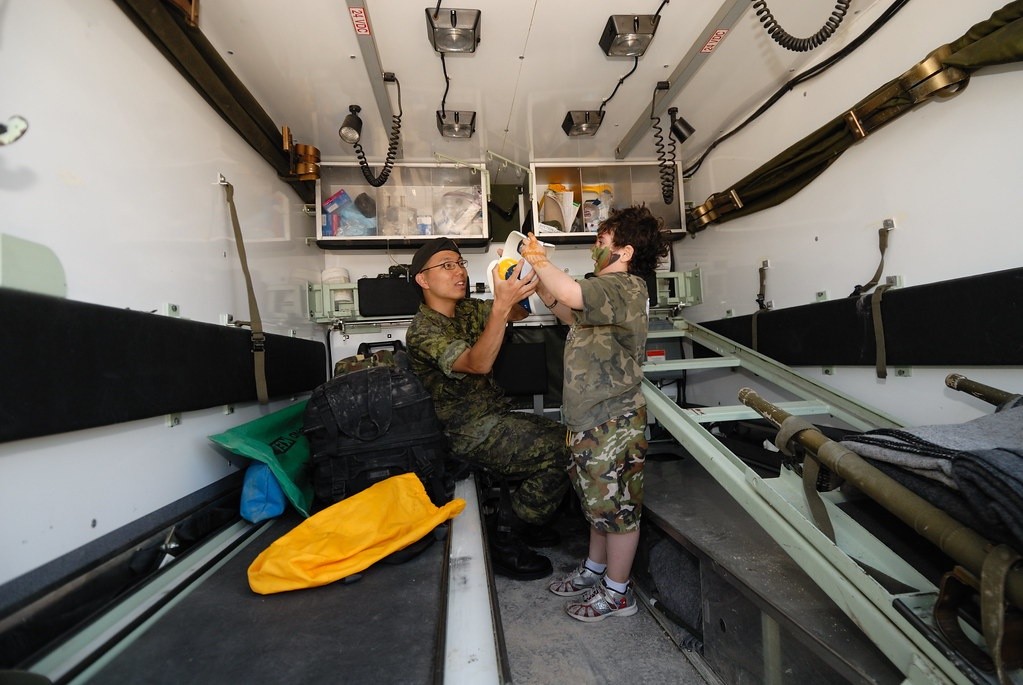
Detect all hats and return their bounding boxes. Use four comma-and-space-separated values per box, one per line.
412, 237, 461, 276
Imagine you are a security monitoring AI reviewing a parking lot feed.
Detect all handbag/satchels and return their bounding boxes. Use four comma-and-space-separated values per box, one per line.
433, 190, 482, 235
245, 472, 467, 594
206, 398, 315, 518
240, 460, 287, 523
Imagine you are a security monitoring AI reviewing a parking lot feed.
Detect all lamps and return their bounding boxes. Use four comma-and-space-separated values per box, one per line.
597, 14, 661, 56
339, 72, 403, 187
649, 81, 696, 204
425, 8, 481, 53
436, 110, 476, 138
561, 110, 606, 137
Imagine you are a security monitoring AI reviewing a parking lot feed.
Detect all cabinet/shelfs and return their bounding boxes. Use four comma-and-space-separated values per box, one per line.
522, 160, 686, 245
314, 161, 489, 250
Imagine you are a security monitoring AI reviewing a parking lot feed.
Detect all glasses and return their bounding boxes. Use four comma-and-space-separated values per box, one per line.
420, 260, 468, 270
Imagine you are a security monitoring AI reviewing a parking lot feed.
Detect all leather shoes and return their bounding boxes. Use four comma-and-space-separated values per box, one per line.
495, 508, 561, 547
485, 512, 553, 581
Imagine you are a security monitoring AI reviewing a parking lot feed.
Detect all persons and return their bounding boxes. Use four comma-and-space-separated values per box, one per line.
406, 238, 571, 579
522, 207, 668, 622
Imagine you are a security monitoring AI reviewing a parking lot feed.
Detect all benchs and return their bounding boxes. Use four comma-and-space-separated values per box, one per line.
0, 288, 513, 685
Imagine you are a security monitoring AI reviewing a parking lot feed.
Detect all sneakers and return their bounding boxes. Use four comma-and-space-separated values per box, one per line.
565, 577, 638, 622
549, 558, 608, 596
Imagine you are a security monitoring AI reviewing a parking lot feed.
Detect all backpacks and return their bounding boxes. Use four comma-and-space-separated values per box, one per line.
334, 350, 392, 376
304, 351, 470, 567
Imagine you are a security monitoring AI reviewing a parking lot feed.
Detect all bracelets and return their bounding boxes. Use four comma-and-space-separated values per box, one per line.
545, 299, 558, 309
